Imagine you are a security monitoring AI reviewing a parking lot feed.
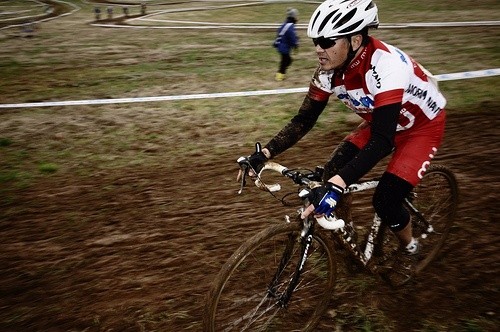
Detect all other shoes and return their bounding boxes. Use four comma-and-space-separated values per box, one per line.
275, 72, 287, 84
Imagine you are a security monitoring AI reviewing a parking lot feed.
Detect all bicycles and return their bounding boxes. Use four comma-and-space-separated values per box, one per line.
203, 141, 459, 332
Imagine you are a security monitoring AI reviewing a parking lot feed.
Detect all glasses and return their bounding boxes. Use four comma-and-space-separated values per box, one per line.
312, 38, 347, 49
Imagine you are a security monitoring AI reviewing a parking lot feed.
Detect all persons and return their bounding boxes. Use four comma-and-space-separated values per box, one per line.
237, 0, 447, 287
273, 7, 301, 81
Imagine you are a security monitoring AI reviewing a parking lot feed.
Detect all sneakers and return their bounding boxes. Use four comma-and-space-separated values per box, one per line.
318, 232, 361, 257
388, 244, 426, 287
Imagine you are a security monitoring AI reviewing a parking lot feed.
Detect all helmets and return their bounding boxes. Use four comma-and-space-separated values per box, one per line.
306, 0, 380, 38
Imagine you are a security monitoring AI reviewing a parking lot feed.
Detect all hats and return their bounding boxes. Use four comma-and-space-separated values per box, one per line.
286, 7, 302, 19
